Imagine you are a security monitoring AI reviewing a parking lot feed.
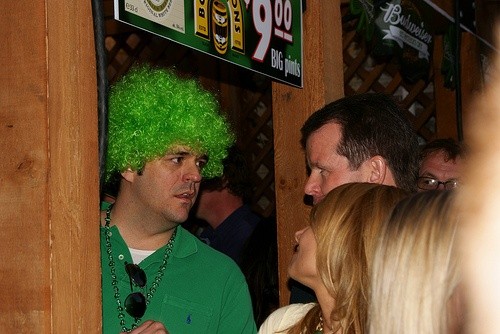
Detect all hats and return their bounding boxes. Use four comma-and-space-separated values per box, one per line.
104, 63, 235, 178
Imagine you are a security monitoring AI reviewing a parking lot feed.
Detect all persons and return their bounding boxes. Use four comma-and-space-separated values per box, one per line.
188, 145, 280, 328
100, 66, 259, 334
257, 64, 500, 334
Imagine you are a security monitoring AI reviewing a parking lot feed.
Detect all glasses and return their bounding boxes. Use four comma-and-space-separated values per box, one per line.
415, 176, 462, 191
123, 261, 147, 323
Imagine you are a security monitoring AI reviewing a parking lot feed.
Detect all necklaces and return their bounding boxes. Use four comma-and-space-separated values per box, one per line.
322, 315, 344, 334
105, 202, 177, 333
